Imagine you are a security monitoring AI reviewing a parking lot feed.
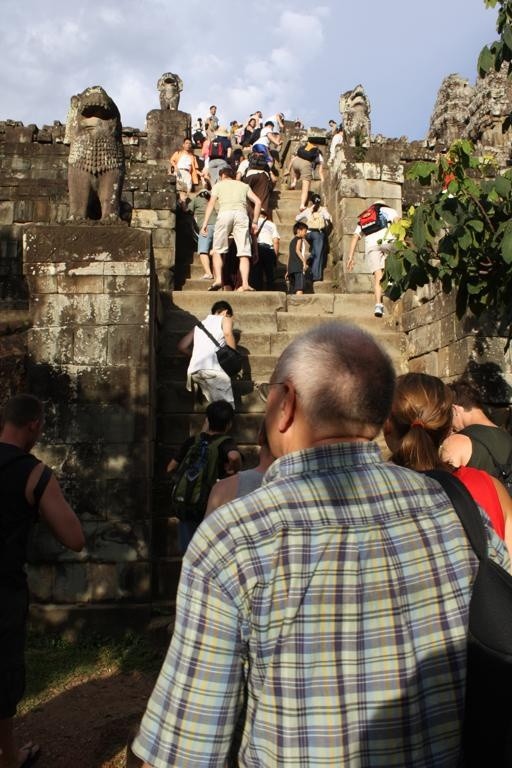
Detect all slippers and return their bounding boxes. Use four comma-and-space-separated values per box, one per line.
208, 283, 222, 291
21, 743, 41, 768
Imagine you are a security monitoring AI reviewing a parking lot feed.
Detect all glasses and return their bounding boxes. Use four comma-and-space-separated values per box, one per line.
255, 383, 280, 403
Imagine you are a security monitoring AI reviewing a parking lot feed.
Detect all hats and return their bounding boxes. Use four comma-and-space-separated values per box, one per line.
214, 125, 230, 137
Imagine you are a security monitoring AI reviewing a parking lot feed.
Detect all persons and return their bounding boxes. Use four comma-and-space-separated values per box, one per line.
131, 317, 512, 768
204, 418, 276, 518
178, 301, 235, 410
170, 105, 344, 295
0, 395, 85, 768
385, 371, 511, 550
440, 380, 511, 488
346, 199, 401, 314
168, 400, 241, 554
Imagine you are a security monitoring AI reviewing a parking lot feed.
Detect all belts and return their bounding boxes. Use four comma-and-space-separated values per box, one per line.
179, 168, 189, 171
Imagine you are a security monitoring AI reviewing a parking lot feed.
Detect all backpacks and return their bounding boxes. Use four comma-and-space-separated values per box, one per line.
297, 142, 323, 161
208, 139, 229, 158
358, 203, 391, 235
247, 152, 270, 172
306, 206, 325, 229
163, 432, 232, 520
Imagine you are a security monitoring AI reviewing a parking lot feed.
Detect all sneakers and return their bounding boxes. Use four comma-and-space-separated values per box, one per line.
374, 303, 384, 314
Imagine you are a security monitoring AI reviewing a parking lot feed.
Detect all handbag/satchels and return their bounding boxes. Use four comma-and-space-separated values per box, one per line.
249, 128, 260, 145
416, 468, 512, 768
216, 345, 242, 377
